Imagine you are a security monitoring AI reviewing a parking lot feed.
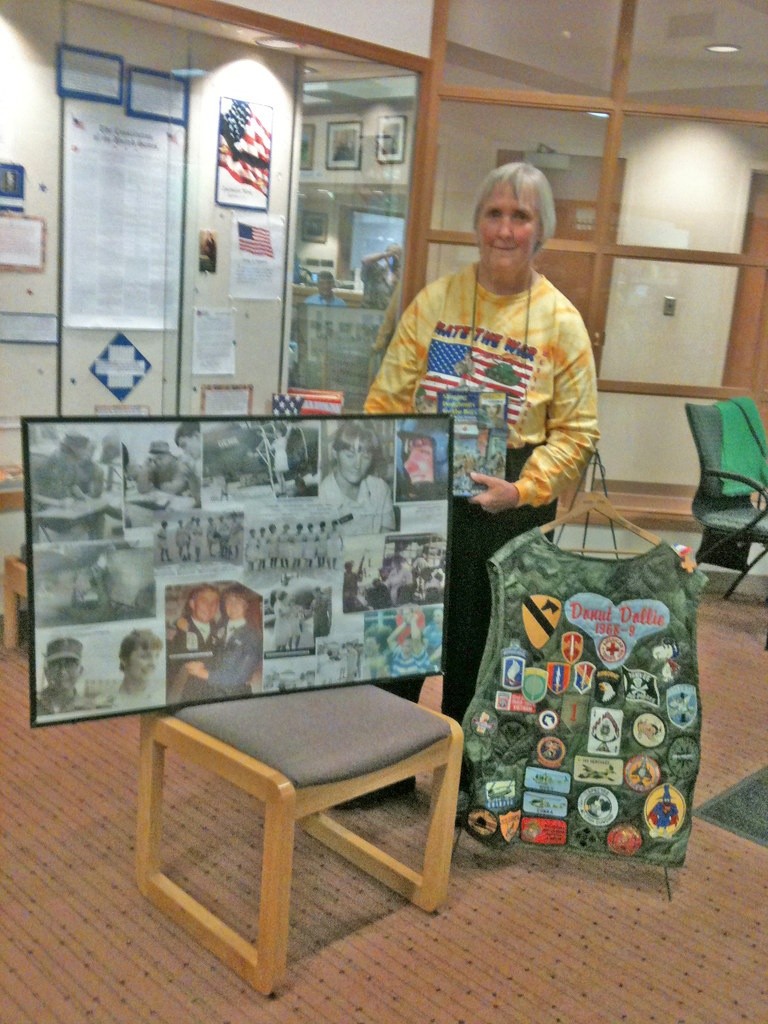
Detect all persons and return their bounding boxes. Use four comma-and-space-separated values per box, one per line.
293, 234, 404, 308
33, 419, 448, 714
332, 161, 598, 826
481, 404, 504, 429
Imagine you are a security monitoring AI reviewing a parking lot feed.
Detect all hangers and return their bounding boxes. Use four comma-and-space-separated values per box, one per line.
539, 462, 662, 556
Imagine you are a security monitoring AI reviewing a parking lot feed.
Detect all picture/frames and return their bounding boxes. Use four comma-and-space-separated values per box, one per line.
376, 113, 407, 165
20, 413, 453, 730
301, 210, 329, 244
300, 121, 315, 170
325, 120, 362, 171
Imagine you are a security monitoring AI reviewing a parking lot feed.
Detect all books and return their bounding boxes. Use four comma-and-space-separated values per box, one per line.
440, 391, 508, 496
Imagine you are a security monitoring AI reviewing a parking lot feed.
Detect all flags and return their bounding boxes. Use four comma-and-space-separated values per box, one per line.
272, 390, 342, 417
220, 100, 269, 197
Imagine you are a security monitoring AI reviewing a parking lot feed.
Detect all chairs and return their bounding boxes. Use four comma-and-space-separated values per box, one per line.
685, 395, 768, 650
134, 684, 466, 997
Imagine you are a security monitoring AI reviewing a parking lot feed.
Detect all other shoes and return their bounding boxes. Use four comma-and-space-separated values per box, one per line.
453, 785, 470, 829
332, 775, 419, 811
